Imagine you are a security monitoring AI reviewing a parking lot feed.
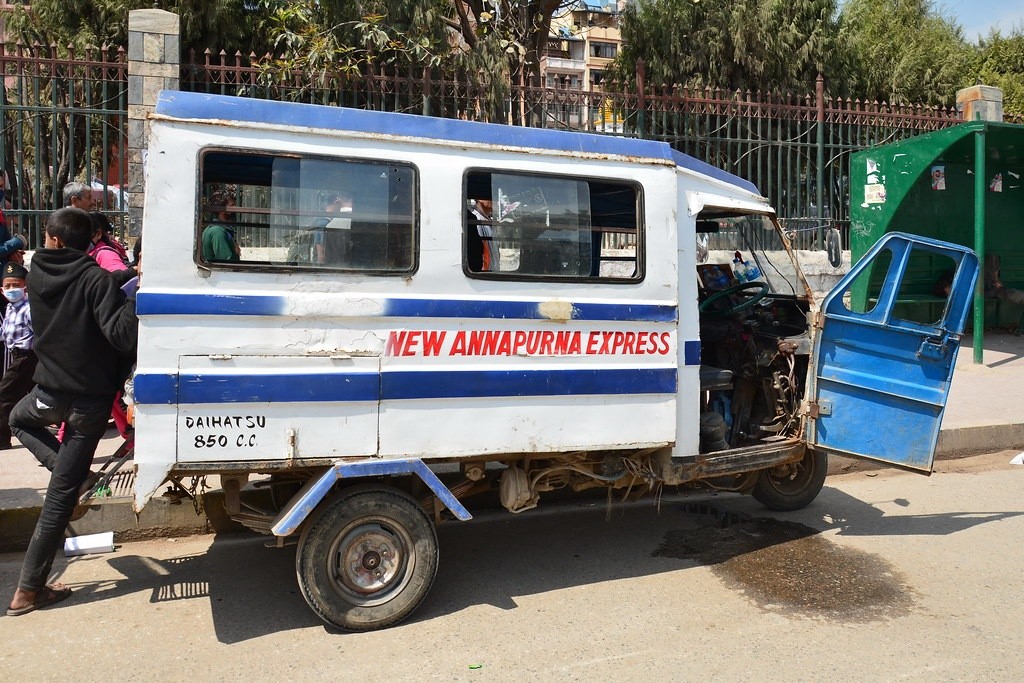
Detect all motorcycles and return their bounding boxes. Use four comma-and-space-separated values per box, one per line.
132, 89, 980, 634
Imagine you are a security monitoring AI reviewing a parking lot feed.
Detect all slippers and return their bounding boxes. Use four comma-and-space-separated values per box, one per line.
67, 470, 100, 521
5, 582, 72, 616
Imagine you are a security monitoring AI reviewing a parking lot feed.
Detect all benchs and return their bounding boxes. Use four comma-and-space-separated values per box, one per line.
869, 251, 1024, 337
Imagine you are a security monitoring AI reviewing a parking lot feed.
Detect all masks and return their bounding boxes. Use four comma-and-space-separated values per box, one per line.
2, 286, 26, 302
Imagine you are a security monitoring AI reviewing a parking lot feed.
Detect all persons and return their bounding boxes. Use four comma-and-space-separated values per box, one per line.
0, 169, 142, 467
3, 207, 141, 616
201, 188, 241, 260
467, 200, 500, 271
305, 191, 353, 265
700, 319, 768, 447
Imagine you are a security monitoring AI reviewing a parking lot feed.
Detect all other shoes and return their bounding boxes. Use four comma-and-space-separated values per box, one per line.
0, 443, 12, 450
118, 448, 129, 457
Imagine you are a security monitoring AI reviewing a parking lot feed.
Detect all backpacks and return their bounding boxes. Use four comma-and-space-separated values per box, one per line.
287, 217, 331, 262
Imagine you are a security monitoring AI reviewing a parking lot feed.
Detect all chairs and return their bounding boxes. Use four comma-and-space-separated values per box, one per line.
701, 366, 734, 392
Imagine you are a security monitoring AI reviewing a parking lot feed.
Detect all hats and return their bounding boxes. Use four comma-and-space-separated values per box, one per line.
2, 262, 28, 280
